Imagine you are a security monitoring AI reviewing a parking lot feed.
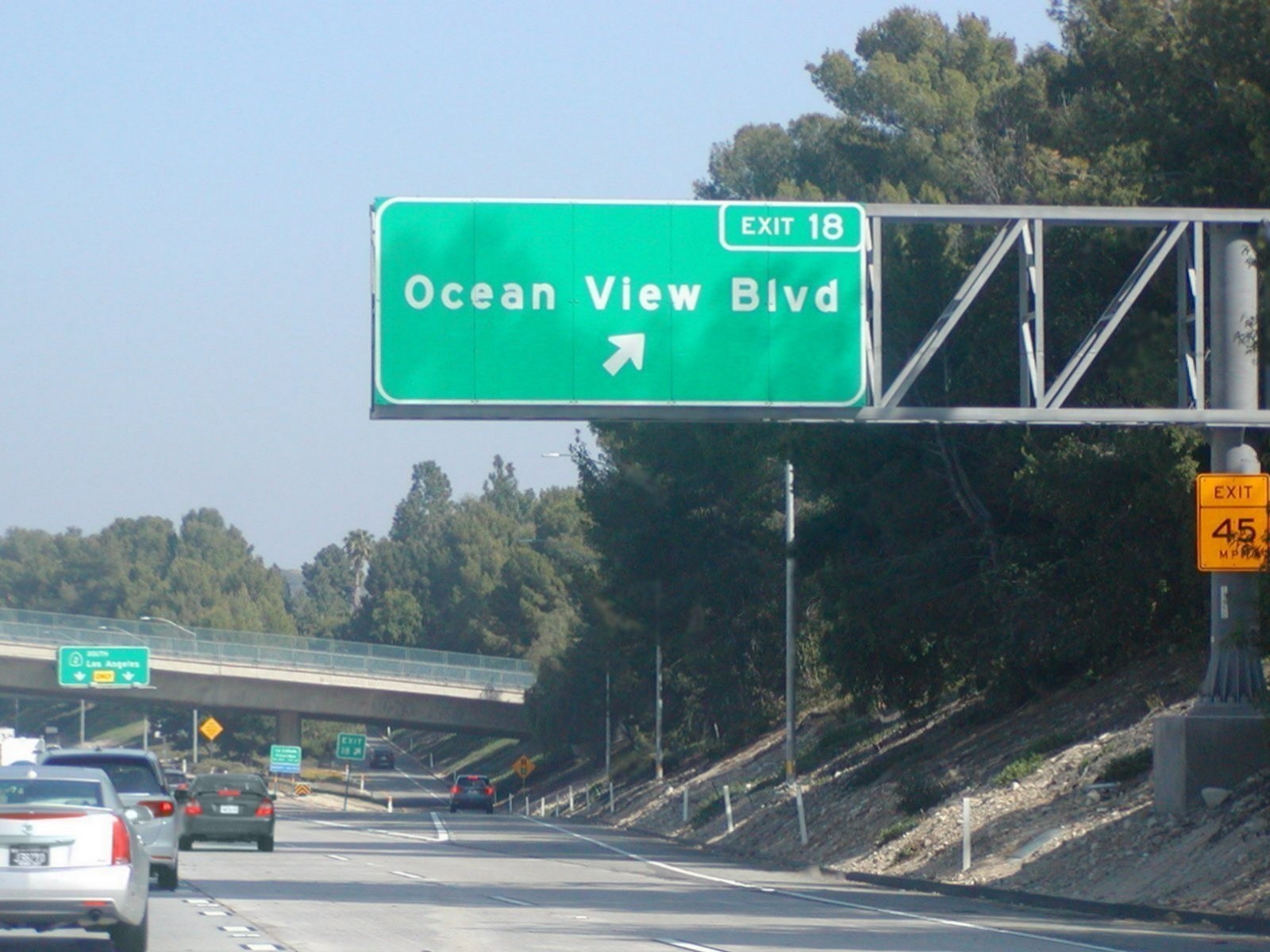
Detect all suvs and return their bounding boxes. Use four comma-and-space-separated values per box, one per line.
35, 746, 192, 892
447, 774, 497, 815
368, 745, 406, 768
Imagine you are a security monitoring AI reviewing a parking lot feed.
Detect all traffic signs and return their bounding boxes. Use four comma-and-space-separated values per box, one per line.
372, 196, 870, 412
336, 732, 366, 761
57, 645, 151, 689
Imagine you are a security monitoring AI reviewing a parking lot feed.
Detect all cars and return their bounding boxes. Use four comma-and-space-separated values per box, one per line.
0, 759, 154, 952
0, 727, 62, 804
171, 772, 278, 854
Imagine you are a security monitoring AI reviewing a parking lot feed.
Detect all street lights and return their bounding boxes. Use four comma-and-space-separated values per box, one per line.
140, 615, 198, 763
99, 625, 148, 751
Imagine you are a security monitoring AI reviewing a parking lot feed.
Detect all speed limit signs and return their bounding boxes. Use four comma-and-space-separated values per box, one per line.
1196, 472, 1270, 570
